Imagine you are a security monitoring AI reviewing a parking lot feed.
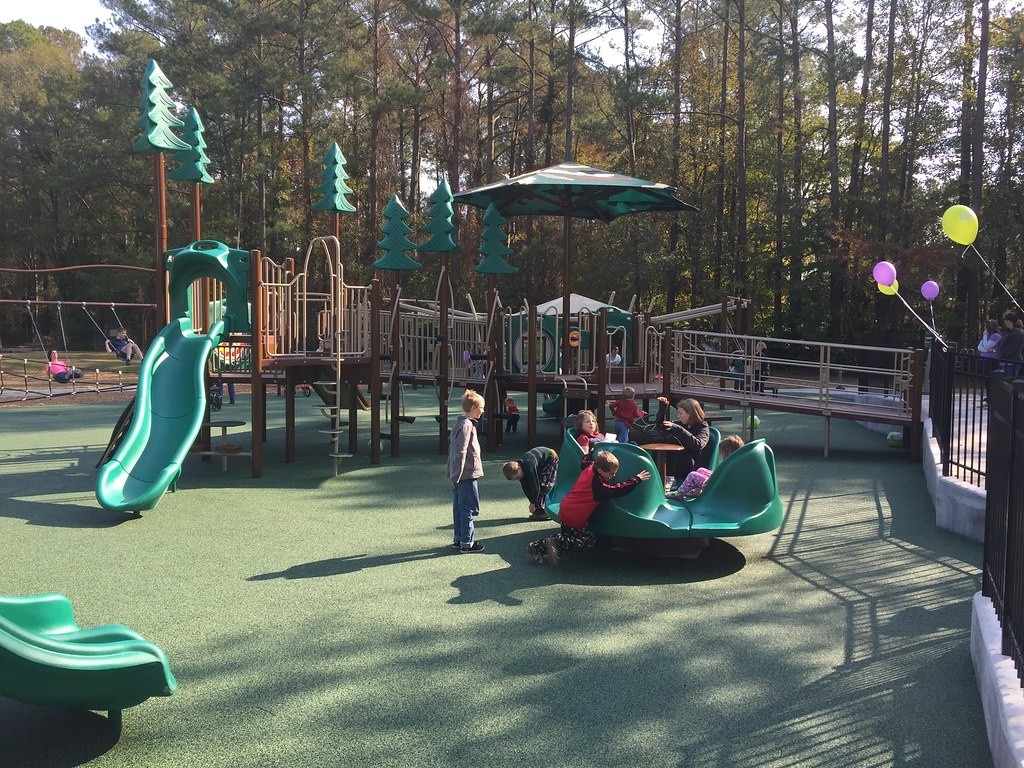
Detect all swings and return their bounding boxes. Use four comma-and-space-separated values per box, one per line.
82, 305, 135, 361
25, 303, 71, 383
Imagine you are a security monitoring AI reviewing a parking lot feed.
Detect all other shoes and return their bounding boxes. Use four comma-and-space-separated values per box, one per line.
65, 371, 72, 379
126, 359, 131, 365
76, 369, 84, 377
528, 546, 545, 564
529, 514, 551, 520
546, 538, 559, 565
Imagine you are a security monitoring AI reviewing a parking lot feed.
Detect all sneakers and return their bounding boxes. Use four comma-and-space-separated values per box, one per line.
460, 541, 484, 553
453, 541, 460, 548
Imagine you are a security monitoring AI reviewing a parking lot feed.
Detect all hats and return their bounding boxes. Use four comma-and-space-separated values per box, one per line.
1002, 313, 1018, 323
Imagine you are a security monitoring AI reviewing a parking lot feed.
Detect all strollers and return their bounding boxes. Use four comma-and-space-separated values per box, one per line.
208, 382, 224, 411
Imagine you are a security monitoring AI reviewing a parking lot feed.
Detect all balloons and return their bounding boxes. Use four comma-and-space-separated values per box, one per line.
873, 261, 898, 295
921, 281, 939, 301
941, 204, 979, 246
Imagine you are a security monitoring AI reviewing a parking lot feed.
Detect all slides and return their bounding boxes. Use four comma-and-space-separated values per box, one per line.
95, 318, 228, 520
310, 382, 371, 411
0, 595, 177, 731
543, 393, 598, 418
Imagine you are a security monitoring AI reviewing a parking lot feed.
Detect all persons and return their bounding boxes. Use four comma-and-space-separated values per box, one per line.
730, 335, 771, 395
105, 328, 145, 366
44, 349, 83, 384
977, 313, 1024, 410
504, 397, 520, 433
657, 397, 709, 491
503, 446, 559, 520
215, 383, 236, 405
528, 452, 651, 564
609, 386, 648, 444
575, 409, 607, 455
315, 340, 325, 352
447, 390, 486, 553
665, 434, 745, 500
606, 345, 621, 367
